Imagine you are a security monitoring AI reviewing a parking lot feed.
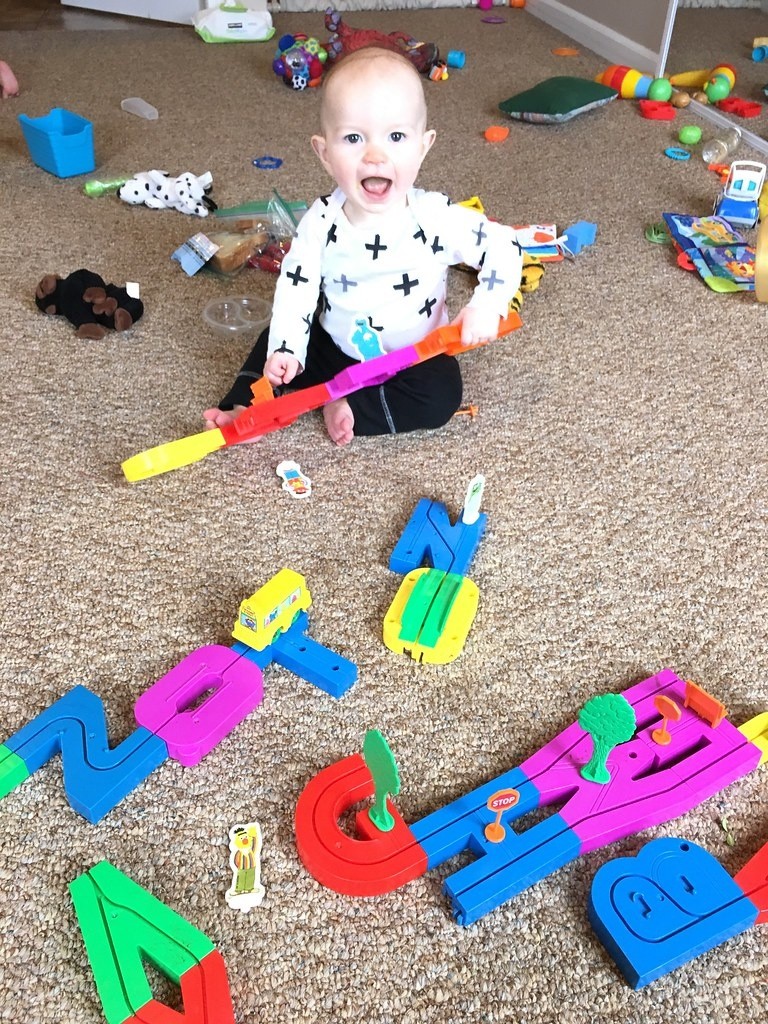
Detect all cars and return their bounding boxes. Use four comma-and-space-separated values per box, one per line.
712, 160, 767, 231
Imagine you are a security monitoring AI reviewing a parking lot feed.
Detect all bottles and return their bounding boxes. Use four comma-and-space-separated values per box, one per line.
702, 126, 741, 164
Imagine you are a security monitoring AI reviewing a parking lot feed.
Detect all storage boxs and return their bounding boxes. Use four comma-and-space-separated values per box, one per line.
17, 107, 95, 179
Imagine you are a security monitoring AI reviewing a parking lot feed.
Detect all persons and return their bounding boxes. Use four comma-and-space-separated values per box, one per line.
202, 46, 524, 449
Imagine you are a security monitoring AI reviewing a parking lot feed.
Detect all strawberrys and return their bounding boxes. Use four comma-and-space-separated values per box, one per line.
247, 238, 291, 273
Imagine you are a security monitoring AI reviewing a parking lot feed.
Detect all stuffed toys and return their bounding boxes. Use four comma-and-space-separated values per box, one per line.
318, 6, 440, 73
34, 268, 145, 341
117, 170, 217, 217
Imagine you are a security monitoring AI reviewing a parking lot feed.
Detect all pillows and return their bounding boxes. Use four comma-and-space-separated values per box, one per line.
497, 73, 619, 124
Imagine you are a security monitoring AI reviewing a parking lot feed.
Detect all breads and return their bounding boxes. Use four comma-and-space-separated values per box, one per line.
209, 218, 270, 272
